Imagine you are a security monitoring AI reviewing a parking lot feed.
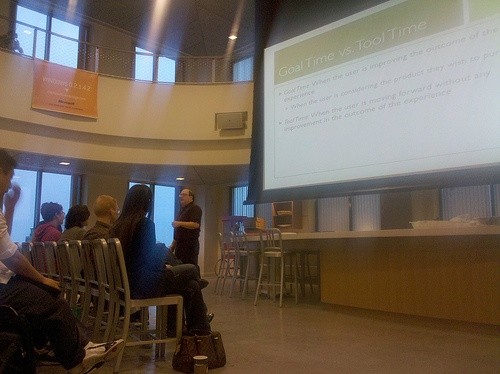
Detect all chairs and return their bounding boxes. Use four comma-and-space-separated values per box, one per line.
13, 238, 183, 374
215, 228, 297, 306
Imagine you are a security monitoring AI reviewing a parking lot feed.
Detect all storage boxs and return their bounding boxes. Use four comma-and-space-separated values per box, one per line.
245, 217, 265, 229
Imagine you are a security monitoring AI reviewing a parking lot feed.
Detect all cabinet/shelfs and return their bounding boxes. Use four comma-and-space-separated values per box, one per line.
271, 200, 302, 229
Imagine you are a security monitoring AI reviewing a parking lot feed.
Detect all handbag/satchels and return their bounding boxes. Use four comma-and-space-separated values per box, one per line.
172, 328, 226, 374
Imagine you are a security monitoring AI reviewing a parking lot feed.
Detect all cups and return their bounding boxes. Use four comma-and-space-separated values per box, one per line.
193, 355, 208, 374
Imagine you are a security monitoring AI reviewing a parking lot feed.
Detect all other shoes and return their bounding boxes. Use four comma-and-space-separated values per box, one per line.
199, 279, 209, 289
208, 312, 214, 323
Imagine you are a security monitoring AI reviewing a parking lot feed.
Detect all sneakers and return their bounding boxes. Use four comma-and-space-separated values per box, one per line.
68, 339, 124, 374
33, 340, 58, 362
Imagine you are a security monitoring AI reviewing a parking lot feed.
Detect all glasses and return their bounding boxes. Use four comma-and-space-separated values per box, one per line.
58, 212, 65, 215
114, 209, 120, 214
179, 194, 190, 198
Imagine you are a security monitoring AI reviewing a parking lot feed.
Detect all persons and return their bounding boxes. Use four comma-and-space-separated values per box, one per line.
169, 188, 203, 266
0, 148, 213, 374
